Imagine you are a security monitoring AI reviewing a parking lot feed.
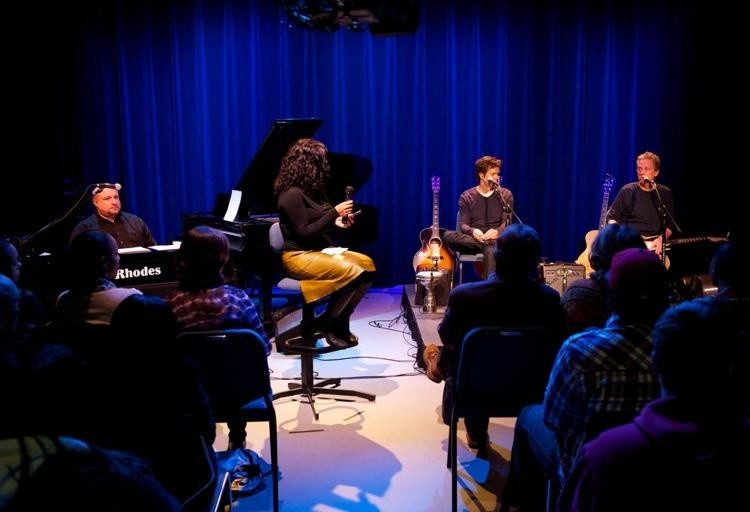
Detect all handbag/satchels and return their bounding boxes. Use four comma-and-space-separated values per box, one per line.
215, 449, 271, 497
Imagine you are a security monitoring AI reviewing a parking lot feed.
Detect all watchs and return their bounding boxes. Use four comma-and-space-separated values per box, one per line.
84, 294, 217, 512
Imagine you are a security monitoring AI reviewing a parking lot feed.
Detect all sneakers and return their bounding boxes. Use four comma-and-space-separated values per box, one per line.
423, 343, 442, 383
466, 431, 488, 447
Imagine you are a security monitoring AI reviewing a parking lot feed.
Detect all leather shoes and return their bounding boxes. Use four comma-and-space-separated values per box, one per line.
316, 318, 357, 348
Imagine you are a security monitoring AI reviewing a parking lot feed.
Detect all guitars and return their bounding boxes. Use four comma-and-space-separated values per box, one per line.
642, 236, 729, 270
412, 175, 459, 275
575, 173, 615, 279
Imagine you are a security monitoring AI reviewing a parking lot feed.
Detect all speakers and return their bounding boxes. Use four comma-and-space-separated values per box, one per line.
678, 273, 719, 297
539, 263, 585, 301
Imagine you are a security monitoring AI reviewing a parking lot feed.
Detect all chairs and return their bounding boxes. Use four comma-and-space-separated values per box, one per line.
173, 327, 273, 476
269, 222, 376, 420
448, 327, 544, 512
3, 445, 235, 510
455, 210, 485, 285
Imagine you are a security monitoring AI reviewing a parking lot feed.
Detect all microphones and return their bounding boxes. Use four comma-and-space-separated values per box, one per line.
342, 186, 353, 224
644, 177, 654, 184
97, 183, 122, 190
487, 178, 499, 186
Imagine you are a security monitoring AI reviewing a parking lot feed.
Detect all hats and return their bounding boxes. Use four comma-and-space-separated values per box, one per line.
609, 248, 665, 296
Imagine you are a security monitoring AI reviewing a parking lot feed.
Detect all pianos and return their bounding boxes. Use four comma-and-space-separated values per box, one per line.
182, 120, 380, 338
27, 241, 183, 290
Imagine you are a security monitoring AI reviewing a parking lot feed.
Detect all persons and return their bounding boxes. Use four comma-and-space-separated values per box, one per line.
559, 222, 651, 329
2, 240, 38, 341
55, 229, 144, 324
437, 224, 560, 478
276, 137, 379, 347
68, 183, 159, 250
707, 239, 749, 308
0, 436, 184, 511
458, 155, 514, 283
0, 273, 36, 436
560, 300, 749, 512
497, 249, 670, 512
605, 151, 673, 272
156, 223, 272, 456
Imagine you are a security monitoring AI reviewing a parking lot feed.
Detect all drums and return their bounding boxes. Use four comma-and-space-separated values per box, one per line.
416, 271, 444, 313
